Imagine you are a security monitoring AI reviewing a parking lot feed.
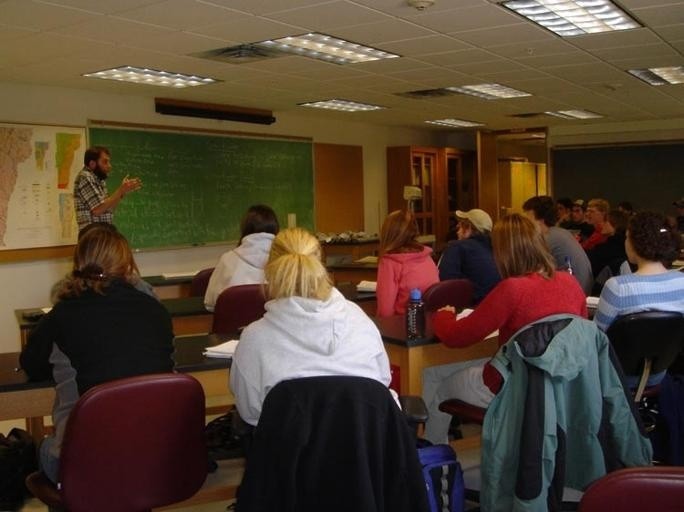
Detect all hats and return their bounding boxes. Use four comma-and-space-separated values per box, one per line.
671, 199, 684, 208
572, 199, 587, 211
455, 208, 493, 235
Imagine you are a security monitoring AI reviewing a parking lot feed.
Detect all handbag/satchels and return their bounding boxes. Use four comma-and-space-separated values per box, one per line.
0, 428, 37, 512
205, 409, 257, 473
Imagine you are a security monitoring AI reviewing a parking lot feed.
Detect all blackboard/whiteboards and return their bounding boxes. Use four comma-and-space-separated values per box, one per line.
547, 139, 684, 217
83, 120, 316, 254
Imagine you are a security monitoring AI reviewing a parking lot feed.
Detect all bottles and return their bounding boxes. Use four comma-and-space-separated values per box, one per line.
558, 256, 576, 276
406, 289, 426, 338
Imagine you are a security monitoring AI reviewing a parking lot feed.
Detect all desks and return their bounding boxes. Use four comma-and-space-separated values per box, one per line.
1, 364, 400, 511
139, 274, 194, 300
318, 236, 379, 258
0, 334, 235, 442
325, 261, 379, 284
14, 297, 213, 353
371, 310, 597, 473
335, 282, 377, 320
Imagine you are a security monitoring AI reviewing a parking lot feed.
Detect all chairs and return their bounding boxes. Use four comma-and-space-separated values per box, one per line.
192, 266, 216, 297
605, 312, 684, 436
233, 375, 428, 510
213, 283, 270, 338
578, 466, 683, 512
26, 373, 208, 511
421, 280, 477, 307
437, 315, 602, 504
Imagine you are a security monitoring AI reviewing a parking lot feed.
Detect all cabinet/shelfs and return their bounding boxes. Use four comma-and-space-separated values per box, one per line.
387, 145, 439, 247
437, 147, 477, 252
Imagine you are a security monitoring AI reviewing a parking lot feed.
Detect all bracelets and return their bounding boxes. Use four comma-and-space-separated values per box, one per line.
120, 195, 124, 199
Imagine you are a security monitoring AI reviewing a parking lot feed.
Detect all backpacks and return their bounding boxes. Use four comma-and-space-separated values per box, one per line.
416, 444, 465, 512
649, 373, 684, 466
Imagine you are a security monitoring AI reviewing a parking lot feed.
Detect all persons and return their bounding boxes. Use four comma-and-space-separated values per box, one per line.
73, 146, 143, 242
421, 212, 589, 444
19, 227, 176, 490
229, 227, 402, 426
376, 209, 441, 318
203, 204, 280, 313
592, 209, 684, 388
77, 221, 158, 299
437, 195, 638, 298
